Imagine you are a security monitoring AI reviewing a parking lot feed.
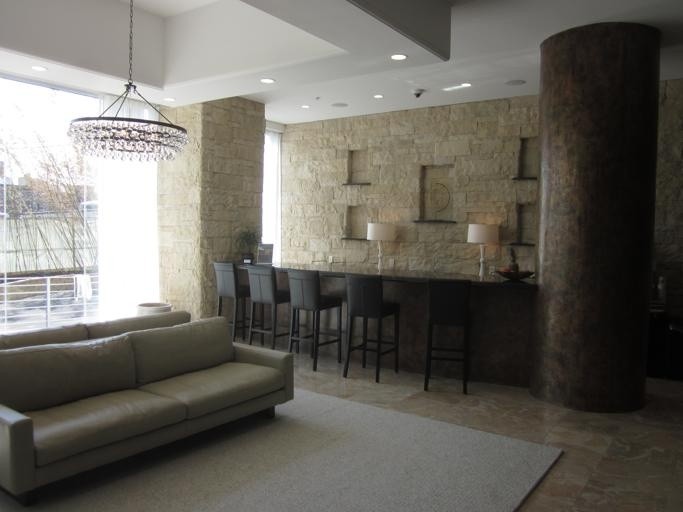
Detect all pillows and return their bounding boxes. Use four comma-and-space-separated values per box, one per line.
0, 335, 137, 414
123, 316, 235, 387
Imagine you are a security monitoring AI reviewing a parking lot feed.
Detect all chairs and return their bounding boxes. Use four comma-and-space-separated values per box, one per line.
288, 268, 341, 372
343, 273, 400, 383
424, 279, 470, 395
247, 266, 300, 354
213, 262, 264, 345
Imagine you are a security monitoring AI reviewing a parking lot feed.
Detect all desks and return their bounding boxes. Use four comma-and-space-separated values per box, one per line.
236, 262, 538, 292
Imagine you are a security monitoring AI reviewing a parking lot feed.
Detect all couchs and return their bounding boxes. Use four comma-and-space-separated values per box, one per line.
0, 310, 294, 498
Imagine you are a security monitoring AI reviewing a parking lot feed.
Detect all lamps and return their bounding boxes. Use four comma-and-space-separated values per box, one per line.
366, 222, 396, 270
66, 0, 187, 163
466, 223, 499, 279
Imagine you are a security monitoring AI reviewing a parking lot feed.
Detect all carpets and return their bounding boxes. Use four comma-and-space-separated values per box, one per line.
0, 385, 563, 511
